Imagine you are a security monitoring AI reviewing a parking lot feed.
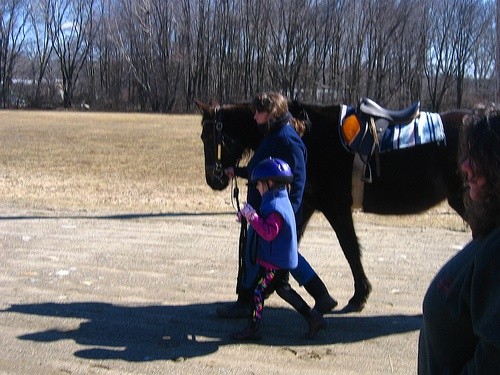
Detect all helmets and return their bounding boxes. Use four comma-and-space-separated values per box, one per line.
250, 158, 293, 183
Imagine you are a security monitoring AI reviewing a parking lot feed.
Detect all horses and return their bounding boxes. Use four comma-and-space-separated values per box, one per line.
193, 97, 472, 318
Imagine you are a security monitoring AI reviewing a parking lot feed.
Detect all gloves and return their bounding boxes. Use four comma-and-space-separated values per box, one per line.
239, 201, 259, 222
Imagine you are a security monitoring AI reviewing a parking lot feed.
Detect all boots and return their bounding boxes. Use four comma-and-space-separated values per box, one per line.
302, 274, 338, 316
304, 309, 330, 338
231, 320, 261, 339
215, 295, 251, 317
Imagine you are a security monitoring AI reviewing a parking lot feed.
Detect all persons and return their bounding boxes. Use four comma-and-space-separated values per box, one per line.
230, 155, 329, 342
214, 87, 338, 322
416, 101, 499, 374
